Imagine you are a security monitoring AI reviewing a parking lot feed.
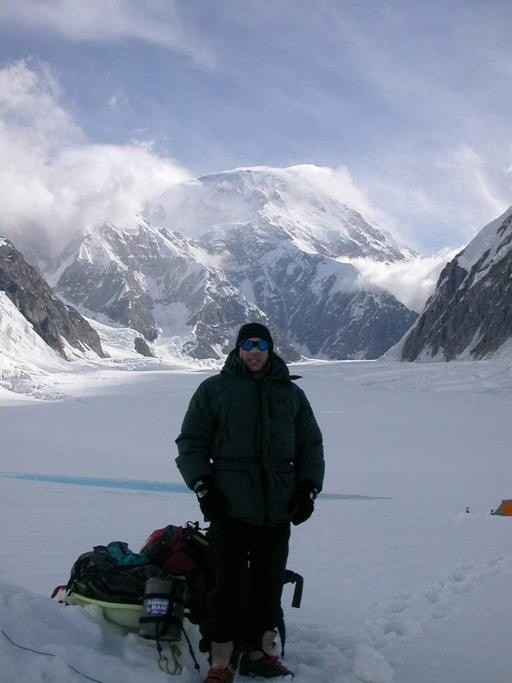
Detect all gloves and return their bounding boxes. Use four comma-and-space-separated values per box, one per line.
195, 483, 215, 519
288, 493, 315, 527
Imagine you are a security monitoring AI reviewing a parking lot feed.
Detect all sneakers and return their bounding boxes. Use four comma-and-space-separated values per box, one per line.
238, 652, 296, 681
203, 666, 235, 683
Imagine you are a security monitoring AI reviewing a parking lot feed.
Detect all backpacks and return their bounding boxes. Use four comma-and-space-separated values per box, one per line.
139, 522, 212, 592
69, 541, 199, 604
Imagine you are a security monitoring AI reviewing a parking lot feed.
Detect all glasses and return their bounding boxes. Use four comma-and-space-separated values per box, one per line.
238, 340, 270, 351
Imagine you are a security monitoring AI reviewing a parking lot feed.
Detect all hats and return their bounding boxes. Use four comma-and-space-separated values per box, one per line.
236, 322, 273, 345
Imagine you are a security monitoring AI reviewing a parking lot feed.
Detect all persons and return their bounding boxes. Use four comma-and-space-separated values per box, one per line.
175, 320, 325, 682
